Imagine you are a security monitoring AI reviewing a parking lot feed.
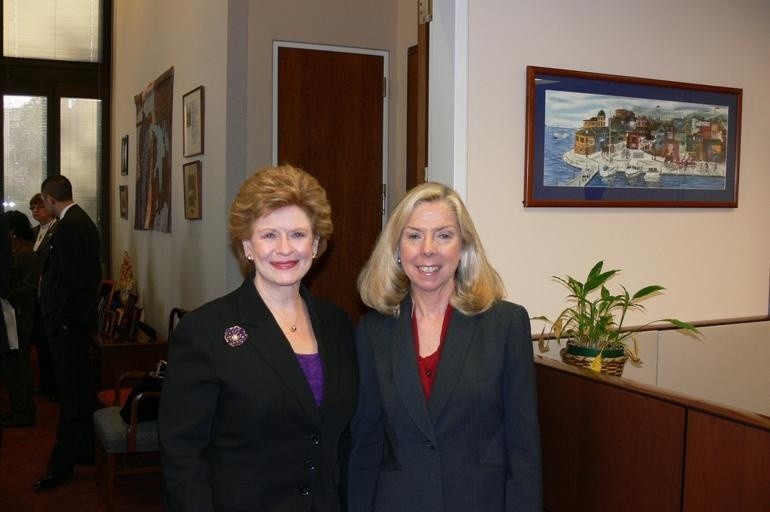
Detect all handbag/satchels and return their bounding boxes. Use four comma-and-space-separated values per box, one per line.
120, 360, 167, 424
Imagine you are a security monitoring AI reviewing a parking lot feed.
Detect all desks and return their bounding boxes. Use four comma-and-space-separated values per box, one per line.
89, 323, 168, 388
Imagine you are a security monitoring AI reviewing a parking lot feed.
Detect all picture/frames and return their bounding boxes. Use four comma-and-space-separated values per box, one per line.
182, 160, 203, 220
182, 85, 205, 158
94, 280, 157, 342
522, 65, 742, 208
118, 184, 128, 221
120, 134, 129, 176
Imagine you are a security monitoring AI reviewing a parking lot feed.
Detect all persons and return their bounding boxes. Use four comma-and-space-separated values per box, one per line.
344, 182, 542, 512
1, 174, 103, 491
156, 160, 359, 512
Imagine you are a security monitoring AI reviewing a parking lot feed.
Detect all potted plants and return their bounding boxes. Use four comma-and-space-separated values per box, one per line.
529, 260, 709, 377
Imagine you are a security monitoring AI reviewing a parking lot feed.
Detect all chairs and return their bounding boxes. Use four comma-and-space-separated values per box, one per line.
95, 308, 189, 408
91, 374, 161, 512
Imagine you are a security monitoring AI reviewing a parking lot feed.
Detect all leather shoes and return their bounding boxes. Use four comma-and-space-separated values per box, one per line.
34, 473, 73, 492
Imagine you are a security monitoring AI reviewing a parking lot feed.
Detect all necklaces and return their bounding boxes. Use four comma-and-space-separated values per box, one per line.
268, 297, 301, 332
